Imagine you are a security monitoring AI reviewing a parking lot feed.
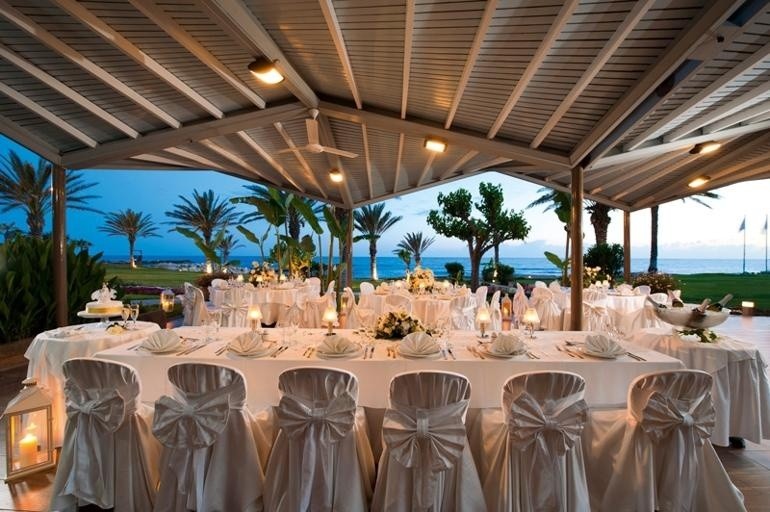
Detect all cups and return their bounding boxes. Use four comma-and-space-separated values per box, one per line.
130, 304, 140, 330
121, 304, 130, 329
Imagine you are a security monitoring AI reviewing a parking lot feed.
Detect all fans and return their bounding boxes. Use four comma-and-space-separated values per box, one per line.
274, 107, 360, 159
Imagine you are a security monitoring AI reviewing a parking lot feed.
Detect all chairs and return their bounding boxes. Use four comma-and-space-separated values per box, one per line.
152, 362, 273, 512
321, 280, 336, 328
590, 368, 748, 512
531, 286, 561, 331
358, 282, 382, 312
626, 293, 668, 332
45, 357, 163, 512
475, 286, 490, 331
211, 279, 228, 288
278, 286, 320, 328
489, 291, 503, 330
370, 370, 488, 512
513, 282, 530, 330
669, 290, 680, 301
385, 295, 412, 314
304, 277, 321, 298
468, 370, 586, 512
344, 287, 375, 329
263, 366, 376, 512
440, 295, 475, 331
634, 286, 650, 295
183, 282, 221, 326
220, 287, 253, 327
582, 289, 623, 331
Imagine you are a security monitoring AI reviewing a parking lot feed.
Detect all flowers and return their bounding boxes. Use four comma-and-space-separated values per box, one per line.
372, 307, 433, 340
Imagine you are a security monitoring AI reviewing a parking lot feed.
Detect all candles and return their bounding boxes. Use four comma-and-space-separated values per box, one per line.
19, 433, 39, 465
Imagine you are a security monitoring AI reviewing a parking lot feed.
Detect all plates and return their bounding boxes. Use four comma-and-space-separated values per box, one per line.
578, 347, 615, 359
397, 348, 443, 358
232, 344, 270, 356
139, 338, 184, 354
478, 343, 528, 358
317, 344, 364, 358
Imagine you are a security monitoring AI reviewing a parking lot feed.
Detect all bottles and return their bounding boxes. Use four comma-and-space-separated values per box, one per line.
646, 295, 666, 308
501, 293, 511, 332
685, 297, 711, 328
707, 294, 733, 312
668, 289, 684, 308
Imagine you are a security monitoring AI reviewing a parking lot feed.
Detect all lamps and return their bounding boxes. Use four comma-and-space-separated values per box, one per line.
247, 59, 285, 86
476, 307, 490, 338
689, 140, 721, 154
322, 306, 337, 336
687, 176, 711, 188
329, 169, 342, 182
523, 308, 540, 339
423, 136, 448, 153
247, 303, 262, 331
